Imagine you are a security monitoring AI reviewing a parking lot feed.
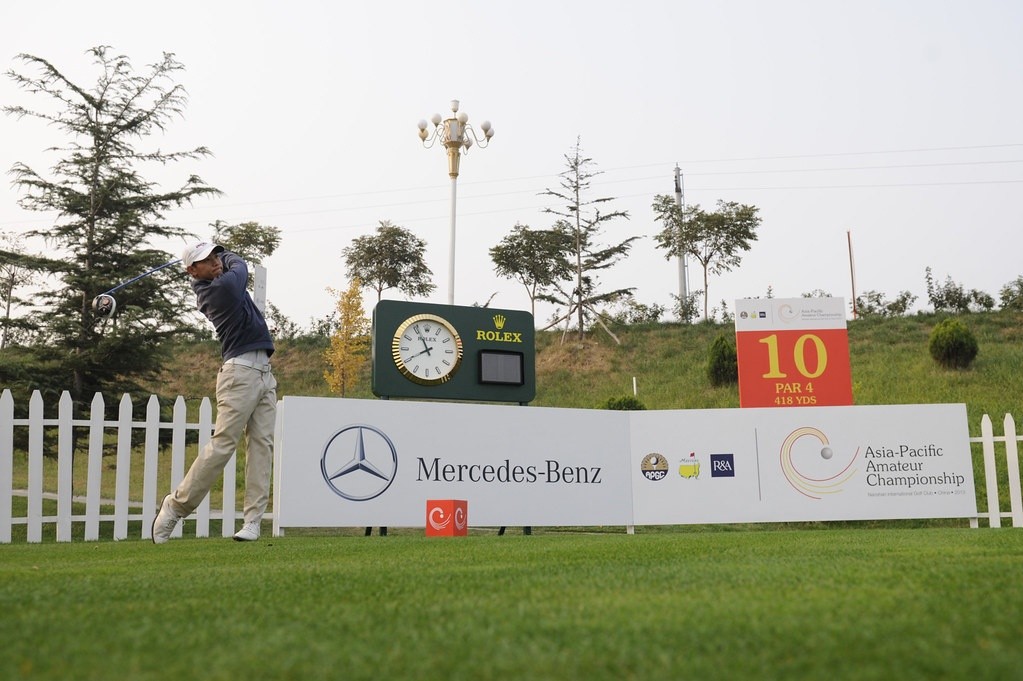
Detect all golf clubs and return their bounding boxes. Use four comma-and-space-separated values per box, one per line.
91, 257, 191, 318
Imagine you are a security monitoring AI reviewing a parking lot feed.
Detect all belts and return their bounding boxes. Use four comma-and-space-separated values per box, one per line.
224, 357, 271, 372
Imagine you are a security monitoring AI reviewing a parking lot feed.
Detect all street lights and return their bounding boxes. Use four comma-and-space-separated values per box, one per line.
418, 99, 495, 305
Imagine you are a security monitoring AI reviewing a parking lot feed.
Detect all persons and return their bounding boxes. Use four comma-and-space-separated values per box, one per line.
151, 241, 277, 544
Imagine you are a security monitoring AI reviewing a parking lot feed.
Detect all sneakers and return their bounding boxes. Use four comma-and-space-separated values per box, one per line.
232, 523, 261, 541
151, 493, 179, 543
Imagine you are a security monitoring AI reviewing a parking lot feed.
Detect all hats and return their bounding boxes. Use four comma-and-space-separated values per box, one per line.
183, 242, 225, 267
